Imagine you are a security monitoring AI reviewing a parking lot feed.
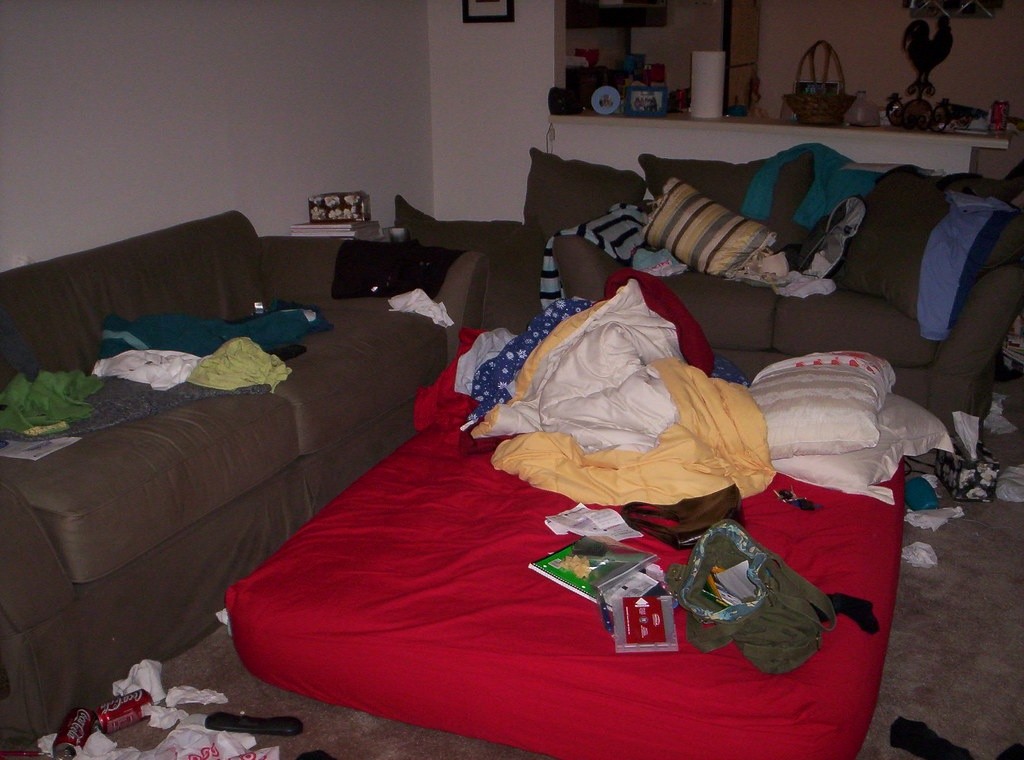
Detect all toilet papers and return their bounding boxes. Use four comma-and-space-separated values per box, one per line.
691, 49, 725, 119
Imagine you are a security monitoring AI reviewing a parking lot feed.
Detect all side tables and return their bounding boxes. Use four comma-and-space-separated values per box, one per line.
290, 219, 380, 240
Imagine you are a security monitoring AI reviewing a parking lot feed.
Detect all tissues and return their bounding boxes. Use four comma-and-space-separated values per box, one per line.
937, 411, 1000, 503
308, 190, 372, 223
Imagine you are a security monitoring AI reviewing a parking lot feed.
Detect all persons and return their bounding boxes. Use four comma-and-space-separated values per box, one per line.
634, 97, 657, 111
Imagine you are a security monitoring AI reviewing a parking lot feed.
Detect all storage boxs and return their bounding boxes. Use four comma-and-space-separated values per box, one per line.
932, 440, 998, 501
309, 190, 371, 222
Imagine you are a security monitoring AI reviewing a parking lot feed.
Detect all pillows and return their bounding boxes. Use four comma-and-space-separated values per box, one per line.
400, 198, 1024, 439
745, 349, 897, 458
394, 147, 1023, 328
771, 394, 949, 490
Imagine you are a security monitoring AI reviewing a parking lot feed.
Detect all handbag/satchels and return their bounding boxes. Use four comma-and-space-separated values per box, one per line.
622, 484, 741, 550
667, 519, 836, 673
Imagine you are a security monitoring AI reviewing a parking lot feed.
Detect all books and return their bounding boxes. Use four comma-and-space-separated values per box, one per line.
527, 535, 646, 612
290, 221, 380, 239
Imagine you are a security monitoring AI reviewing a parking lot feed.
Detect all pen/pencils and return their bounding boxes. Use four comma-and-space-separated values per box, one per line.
599, 595, 612, 632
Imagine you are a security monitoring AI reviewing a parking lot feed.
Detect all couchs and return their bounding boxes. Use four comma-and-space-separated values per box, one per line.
0, 209, 489, 759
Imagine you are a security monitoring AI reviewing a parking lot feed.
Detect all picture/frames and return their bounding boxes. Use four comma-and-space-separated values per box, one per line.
624, 84, 670, 118
462, 0, 516, 23
792, 79, 843, 120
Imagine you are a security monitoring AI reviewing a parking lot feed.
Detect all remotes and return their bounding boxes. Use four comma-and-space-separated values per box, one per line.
204, 711, 304, 737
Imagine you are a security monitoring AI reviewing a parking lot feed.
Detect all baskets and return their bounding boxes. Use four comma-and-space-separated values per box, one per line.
784, 40, 857, 123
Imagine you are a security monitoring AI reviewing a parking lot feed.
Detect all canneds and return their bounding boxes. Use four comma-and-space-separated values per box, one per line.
53, 707, 98, 760
675, 89, 686, 109
988, 99, 1009, 133
94, 688, 154, 734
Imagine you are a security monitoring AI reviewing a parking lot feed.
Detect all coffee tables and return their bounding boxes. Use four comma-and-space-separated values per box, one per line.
225, 391, 907, 759
